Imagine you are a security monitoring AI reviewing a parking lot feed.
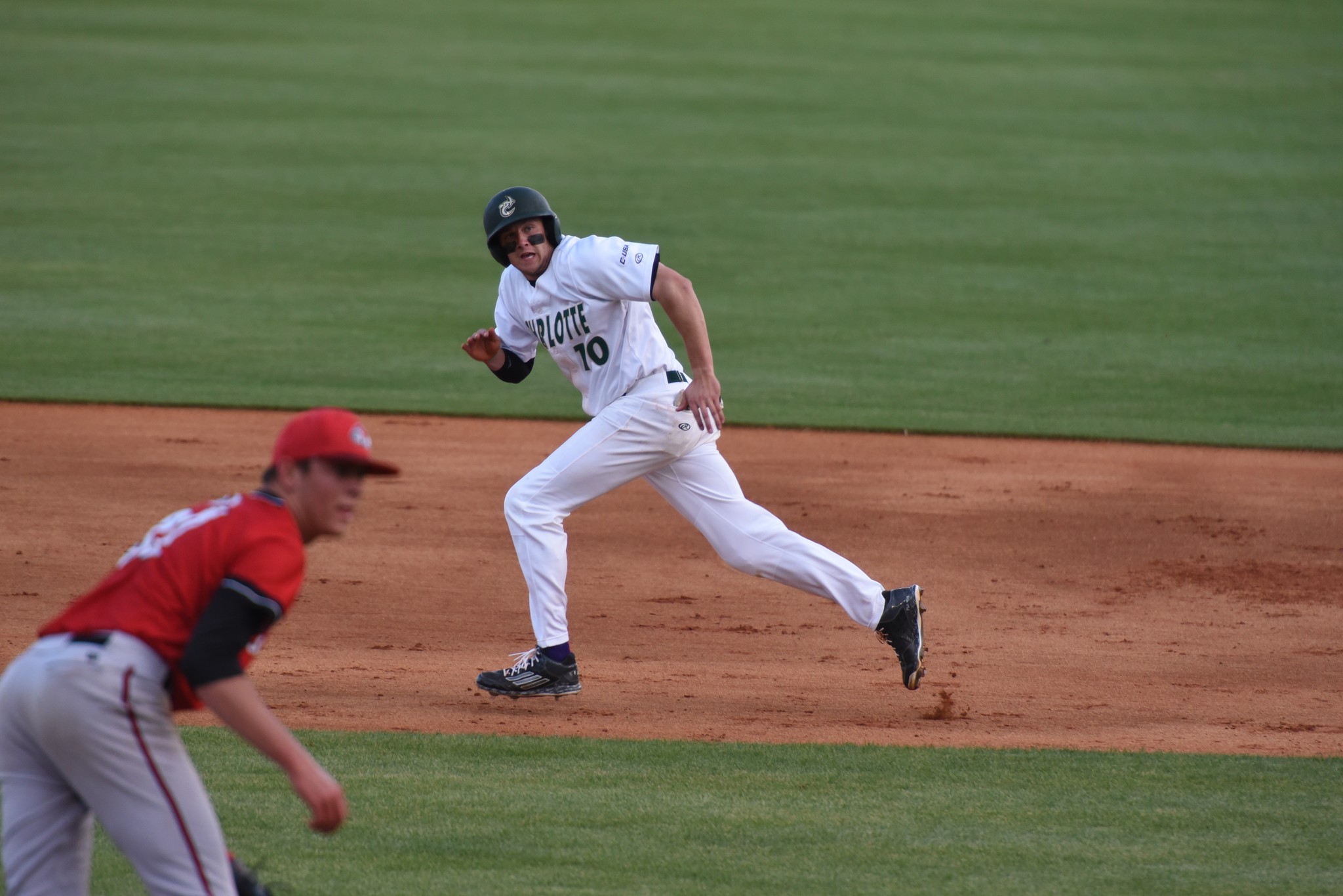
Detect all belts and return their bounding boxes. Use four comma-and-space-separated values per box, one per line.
666, 370, 689, 384
71, 632, 107, 648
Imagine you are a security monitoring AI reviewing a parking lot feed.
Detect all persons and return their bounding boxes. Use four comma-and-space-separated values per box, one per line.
458, 188, 931, 699
0, 407, 404, 896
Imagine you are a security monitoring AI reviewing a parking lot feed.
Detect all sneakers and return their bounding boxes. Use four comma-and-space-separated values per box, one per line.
475, 644, 581, 700
873, 583, 927, 690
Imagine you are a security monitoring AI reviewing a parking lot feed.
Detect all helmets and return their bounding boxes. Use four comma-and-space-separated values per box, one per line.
483, 187, 563, 268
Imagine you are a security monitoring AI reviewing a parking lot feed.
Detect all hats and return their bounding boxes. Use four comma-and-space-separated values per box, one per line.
275, 407, 398, 475
